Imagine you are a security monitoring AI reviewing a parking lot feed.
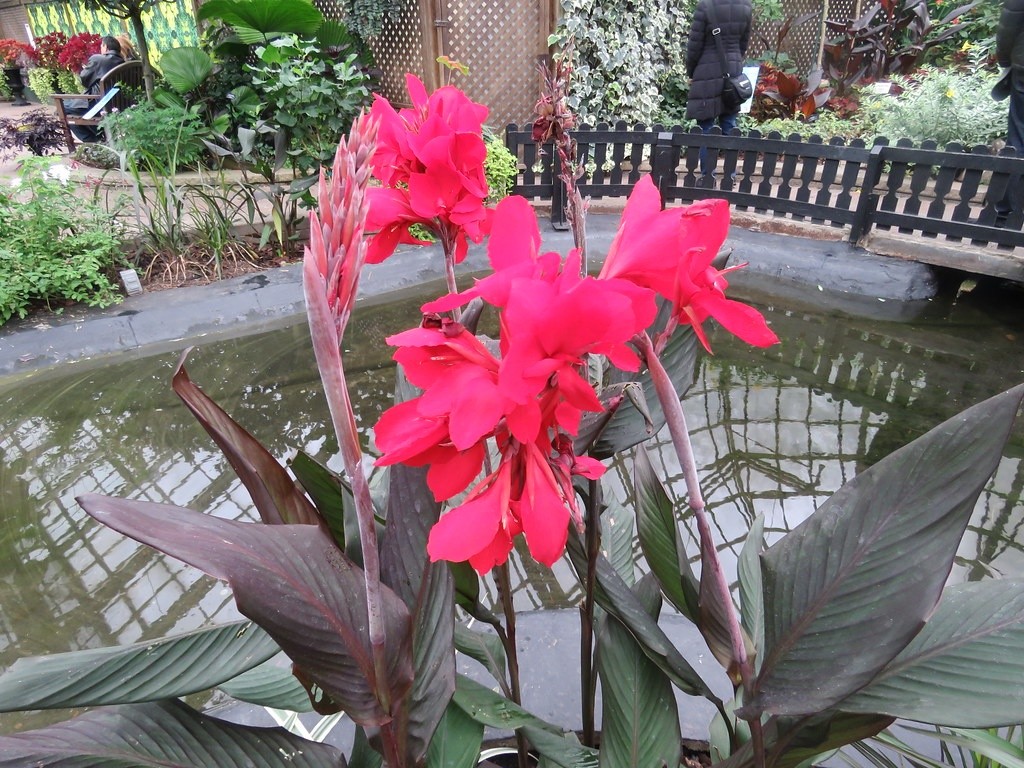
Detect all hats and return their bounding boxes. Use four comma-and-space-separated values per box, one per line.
991, 66, 1011, 104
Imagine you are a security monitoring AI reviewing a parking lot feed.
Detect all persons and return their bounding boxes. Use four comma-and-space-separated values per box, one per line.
962, 0, 1024, 240
53, 34, 143, 143
685, 0, 753, 190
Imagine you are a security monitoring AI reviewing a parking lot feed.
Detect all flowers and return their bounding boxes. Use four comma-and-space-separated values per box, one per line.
0, 53, 1024, 768
0, 30, 103, 73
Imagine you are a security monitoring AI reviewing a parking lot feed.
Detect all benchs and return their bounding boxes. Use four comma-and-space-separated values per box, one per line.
47, 59, 164, 155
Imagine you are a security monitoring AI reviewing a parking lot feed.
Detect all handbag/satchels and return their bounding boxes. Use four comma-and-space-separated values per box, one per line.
725, 73, 753, 107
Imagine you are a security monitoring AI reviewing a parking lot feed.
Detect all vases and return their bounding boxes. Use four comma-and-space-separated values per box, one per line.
3, 66, 30, 108
